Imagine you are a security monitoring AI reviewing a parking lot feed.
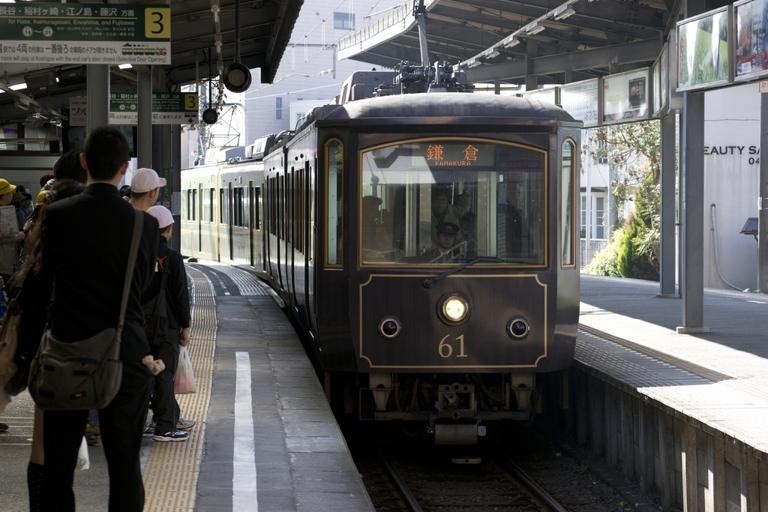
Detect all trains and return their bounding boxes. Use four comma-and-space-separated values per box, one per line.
181, 58, 591, 478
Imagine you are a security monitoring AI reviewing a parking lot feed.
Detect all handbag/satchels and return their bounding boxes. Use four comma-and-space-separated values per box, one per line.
26, 331, 122, 411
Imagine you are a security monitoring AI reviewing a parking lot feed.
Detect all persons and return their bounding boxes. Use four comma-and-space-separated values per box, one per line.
0, 152, 86, 435
119, 169, 197, 443
361, 181, 522, 259
11, 122, 166, 512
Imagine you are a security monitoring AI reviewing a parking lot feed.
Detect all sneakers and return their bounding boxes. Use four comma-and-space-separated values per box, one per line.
143, 417, 195, 442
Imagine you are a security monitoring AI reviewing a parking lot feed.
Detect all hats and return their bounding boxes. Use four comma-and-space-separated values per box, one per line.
35, 190, 54, 206
436, 222, 459, 235
130, 167, 167, 192
145, 206, 175, 228
364, 196, 383, 212
454, 192, 470, 207
0, 178, 16, 195
